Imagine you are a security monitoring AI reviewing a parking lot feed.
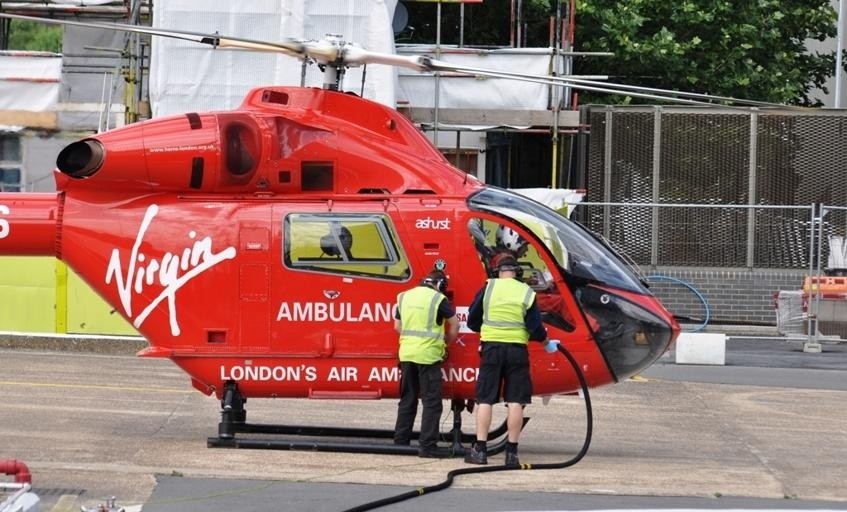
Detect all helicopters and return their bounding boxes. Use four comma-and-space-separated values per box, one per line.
0, 8, 823, 460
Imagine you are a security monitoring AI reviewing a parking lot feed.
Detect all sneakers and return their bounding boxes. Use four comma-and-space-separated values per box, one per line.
419, 447, 450, 457
465, 450, 487, 464
505, 452, 519, 466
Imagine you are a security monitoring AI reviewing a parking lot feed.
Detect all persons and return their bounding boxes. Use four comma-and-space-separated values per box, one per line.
393, 270, 459, 447
488, 223, 531, 259
463, 253, 563, 464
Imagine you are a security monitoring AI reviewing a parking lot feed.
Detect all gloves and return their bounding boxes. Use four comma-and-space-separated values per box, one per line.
544, 339, 560, 353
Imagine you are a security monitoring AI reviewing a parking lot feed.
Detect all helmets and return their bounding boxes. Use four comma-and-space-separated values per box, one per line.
495, 224, 528, 252
